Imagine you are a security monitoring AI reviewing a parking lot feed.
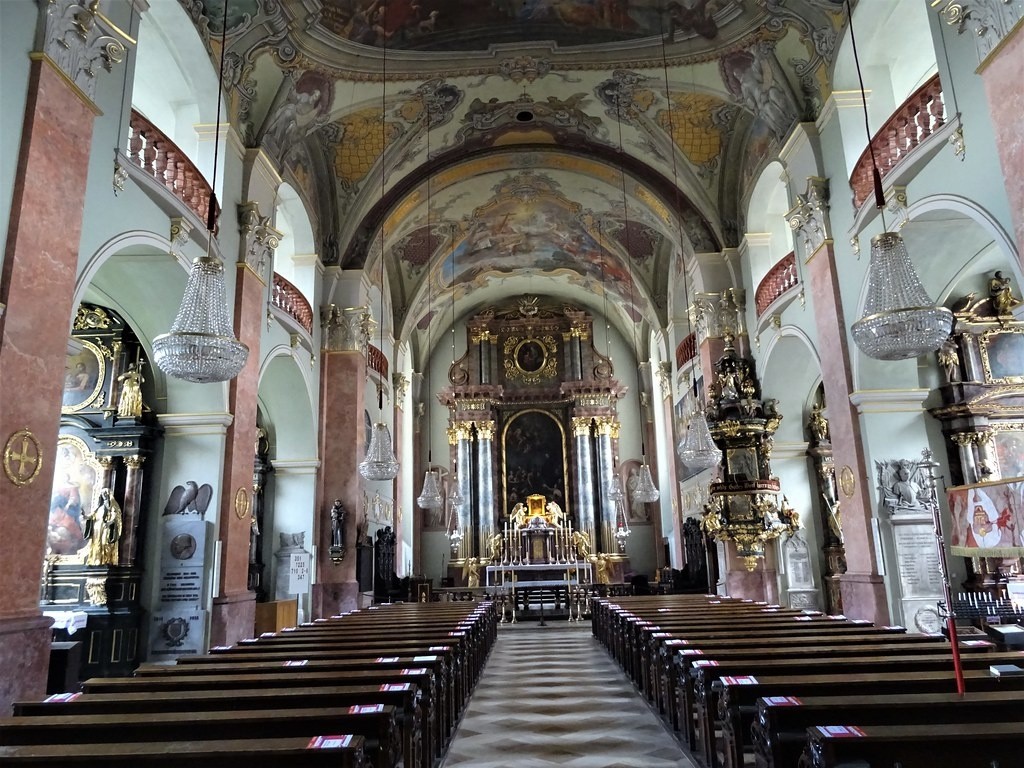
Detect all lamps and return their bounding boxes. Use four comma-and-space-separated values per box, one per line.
615, 89, 660, 504
415, 90, 446, 509
359, 2, 400, 483
599, 220, 633, 551
152, 2, 250, 383
597, 218, 627, 500
444, 224, 466, 553
699, 287, 801, 571
843, 0, 953, 363
658, 2, 725, 471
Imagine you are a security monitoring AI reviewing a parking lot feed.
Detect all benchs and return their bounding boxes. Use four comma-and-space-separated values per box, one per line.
590, 593, 1024, 768
0, 599, 509, 768
